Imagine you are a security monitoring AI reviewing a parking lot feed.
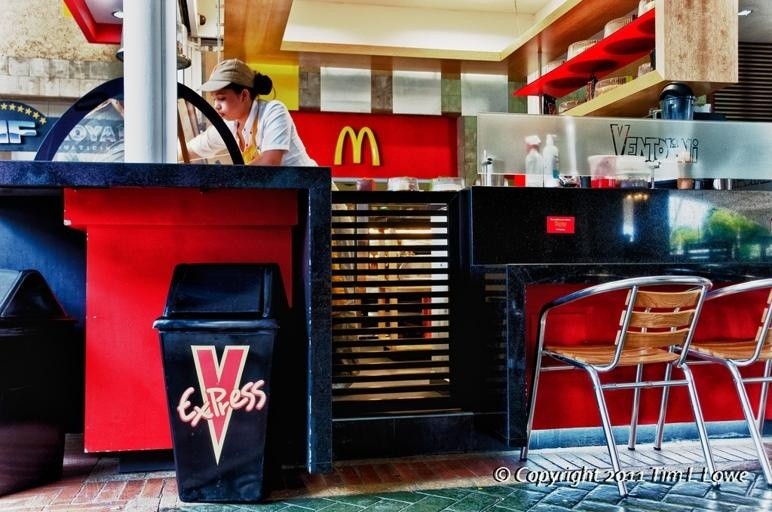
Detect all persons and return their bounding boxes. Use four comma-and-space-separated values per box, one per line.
178, 59, 362, 395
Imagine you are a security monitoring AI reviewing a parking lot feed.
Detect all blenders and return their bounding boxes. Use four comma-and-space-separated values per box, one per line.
650, 81, 699, 190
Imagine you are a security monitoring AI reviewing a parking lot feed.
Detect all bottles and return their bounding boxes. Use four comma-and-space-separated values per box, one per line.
480, 157, 504, 188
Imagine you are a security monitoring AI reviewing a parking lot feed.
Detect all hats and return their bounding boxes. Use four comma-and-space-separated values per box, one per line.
196, 57, 255, 93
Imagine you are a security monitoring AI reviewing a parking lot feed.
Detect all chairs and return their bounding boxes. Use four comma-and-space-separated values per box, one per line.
520, 274, 770, 496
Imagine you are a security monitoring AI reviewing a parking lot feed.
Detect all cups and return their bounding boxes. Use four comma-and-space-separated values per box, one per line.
587, 155, 651, 189
714, 178, 733, 190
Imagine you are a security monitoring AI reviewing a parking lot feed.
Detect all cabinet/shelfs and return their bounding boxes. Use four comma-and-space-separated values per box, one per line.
512, 0, 737, 117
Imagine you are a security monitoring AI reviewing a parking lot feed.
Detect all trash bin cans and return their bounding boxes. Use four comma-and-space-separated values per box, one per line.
0, 266, 78, 497
152, 262, 294, 504
